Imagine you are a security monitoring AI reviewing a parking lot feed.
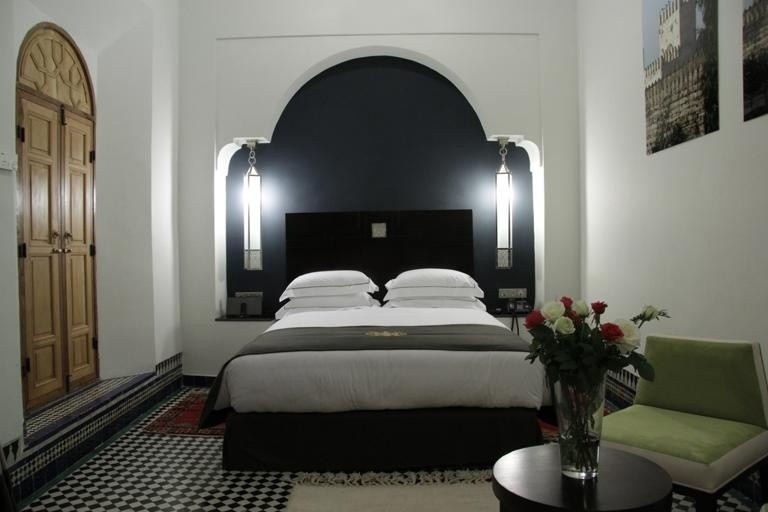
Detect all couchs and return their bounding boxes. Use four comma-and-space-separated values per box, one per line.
601, 337, 767, 511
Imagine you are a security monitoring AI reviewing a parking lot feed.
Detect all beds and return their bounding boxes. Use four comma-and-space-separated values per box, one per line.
197, 211, 557, 476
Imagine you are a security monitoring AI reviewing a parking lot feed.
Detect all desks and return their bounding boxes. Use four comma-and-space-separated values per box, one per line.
490, 441, 675, 512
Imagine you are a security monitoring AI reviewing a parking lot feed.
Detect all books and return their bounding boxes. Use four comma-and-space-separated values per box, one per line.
228, 295, 263, 317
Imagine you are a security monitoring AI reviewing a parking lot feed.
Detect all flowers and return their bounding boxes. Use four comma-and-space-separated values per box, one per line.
525, 297, 673, 471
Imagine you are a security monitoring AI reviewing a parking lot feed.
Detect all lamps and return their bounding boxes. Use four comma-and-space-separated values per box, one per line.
243, 140, 263, 271
494, 138, 515, 271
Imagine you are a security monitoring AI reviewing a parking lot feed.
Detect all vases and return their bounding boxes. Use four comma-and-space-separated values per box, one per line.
549, 377, 608, 485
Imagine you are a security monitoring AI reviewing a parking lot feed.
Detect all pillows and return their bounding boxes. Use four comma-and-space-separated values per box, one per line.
278, 268, 380, 303
273, 295, 381, 321
382, 267, 487, 302
382, 294, 487, 312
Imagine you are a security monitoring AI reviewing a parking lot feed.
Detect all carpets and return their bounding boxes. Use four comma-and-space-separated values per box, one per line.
285, 466, 504, 512
